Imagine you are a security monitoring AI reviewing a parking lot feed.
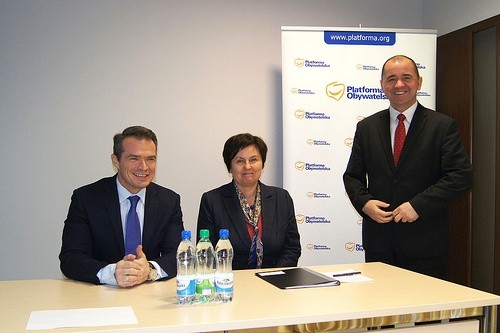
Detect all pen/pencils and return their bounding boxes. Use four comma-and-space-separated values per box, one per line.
333, 271, 361, 277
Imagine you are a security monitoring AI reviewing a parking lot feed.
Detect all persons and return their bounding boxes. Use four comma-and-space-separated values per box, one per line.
196, 133, 302, 270
59, 126, 185, 288
343, 56, 472, 279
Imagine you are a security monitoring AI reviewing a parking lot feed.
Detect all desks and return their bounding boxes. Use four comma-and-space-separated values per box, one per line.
0, 261, 500, 333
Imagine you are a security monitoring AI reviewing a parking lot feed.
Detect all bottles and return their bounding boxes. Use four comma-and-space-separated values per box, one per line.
196, 229, 215, 304
215, 229, 234, 303
176, 230, 196, 305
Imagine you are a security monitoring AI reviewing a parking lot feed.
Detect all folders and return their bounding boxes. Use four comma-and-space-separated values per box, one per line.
255, 268, 340, 289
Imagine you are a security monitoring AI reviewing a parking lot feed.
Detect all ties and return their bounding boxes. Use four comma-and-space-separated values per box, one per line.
125, 196, 141, 257
394, 114, 406, 168
